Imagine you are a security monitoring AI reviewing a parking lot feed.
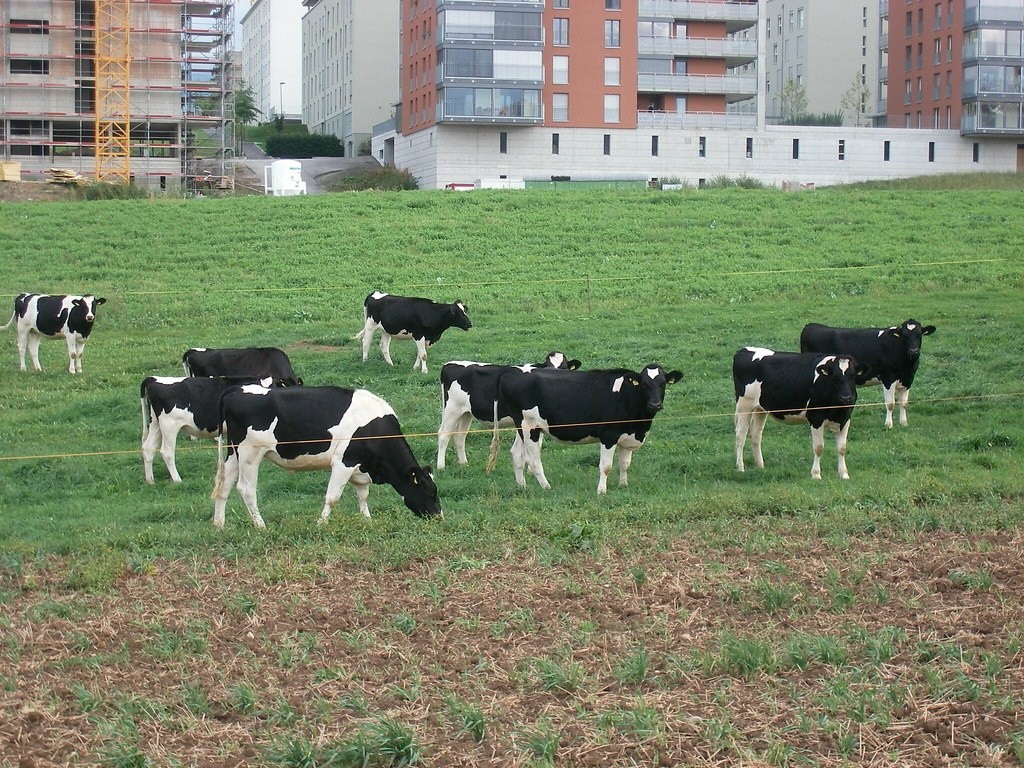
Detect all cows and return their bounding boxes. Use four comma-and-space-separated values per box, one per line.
734, 319, 936, 479
1, 294, 107, 374
436, 351, 683, 495
348, 291, 472, 373
141, 346, 444, 533
